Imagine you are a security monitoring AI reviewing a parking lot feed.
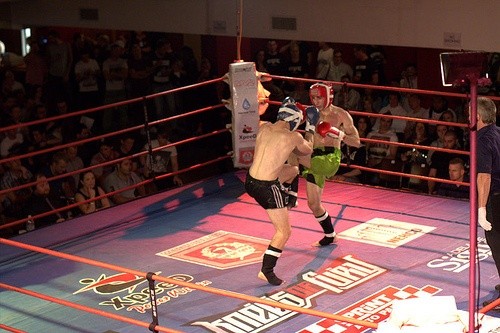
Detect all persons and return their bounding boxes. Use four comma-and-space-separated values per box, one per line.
437, 159, 470, 198
245, 97, 321, 286
23, 173, 83, 229
256, 39, 500, 193
286, 82, 361, 246
102, 155, 145, 205
468, 96, 500, 310
74, 170, 110, 215
0, 31, 233, 202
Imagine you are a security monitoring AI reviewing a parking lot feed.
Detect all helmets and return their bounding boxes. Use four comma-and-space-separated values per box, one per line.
278, 101, 307, 131
309, 82, 334, 110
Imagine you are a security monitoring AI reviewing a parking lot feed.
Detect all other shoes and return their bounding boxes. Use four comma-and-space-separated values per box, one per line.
483, 295, 500, 310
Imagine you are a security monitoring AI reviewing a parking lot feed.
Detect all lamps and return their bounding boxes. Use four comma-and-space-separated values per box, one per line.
0, 26, 31, 57
439, 51, 490, 88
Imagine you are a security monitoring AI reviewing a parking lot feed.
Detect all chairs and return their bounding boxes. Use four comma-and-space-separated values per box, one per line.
375, 156, 405, 190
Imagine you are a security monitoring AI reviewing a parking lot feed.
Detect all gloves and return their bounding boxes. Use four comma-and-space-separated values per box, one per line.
294, 102, 307, 125
316, 120, 345, 141
477, 207, 493, 232
306, 106, 320, 134
282, 96, 295, 104
